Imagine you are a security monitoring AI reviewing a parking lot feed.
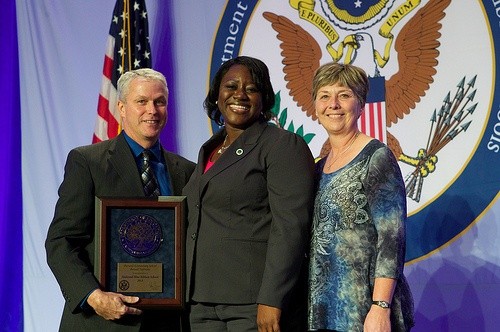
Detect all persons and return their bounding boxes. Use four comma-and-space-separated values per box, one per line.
44, 69, 196, 332
180, 56, 317, 332
298, 62, 415, 332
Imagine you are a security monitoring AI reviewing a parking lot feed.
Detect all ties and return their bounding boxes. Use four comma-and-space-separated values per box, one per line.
140, 149, 159, 200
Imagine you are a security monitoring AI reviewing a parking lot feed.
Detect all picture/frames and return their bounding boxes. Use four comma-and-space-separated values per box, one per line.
94, 196, 187, 307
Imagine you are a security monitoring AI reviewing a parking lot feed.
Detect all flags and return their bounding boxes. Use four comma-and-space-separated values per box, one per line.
92, 0, 152, 143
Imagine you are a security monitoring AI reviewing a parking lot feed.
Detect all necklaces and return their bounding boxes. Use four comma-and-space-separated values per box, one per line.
218, 134, 232, 154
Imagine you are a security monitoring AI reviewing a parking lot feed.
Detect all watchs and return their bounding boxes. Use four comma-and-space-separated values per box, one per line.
371, 301, 392, 308
